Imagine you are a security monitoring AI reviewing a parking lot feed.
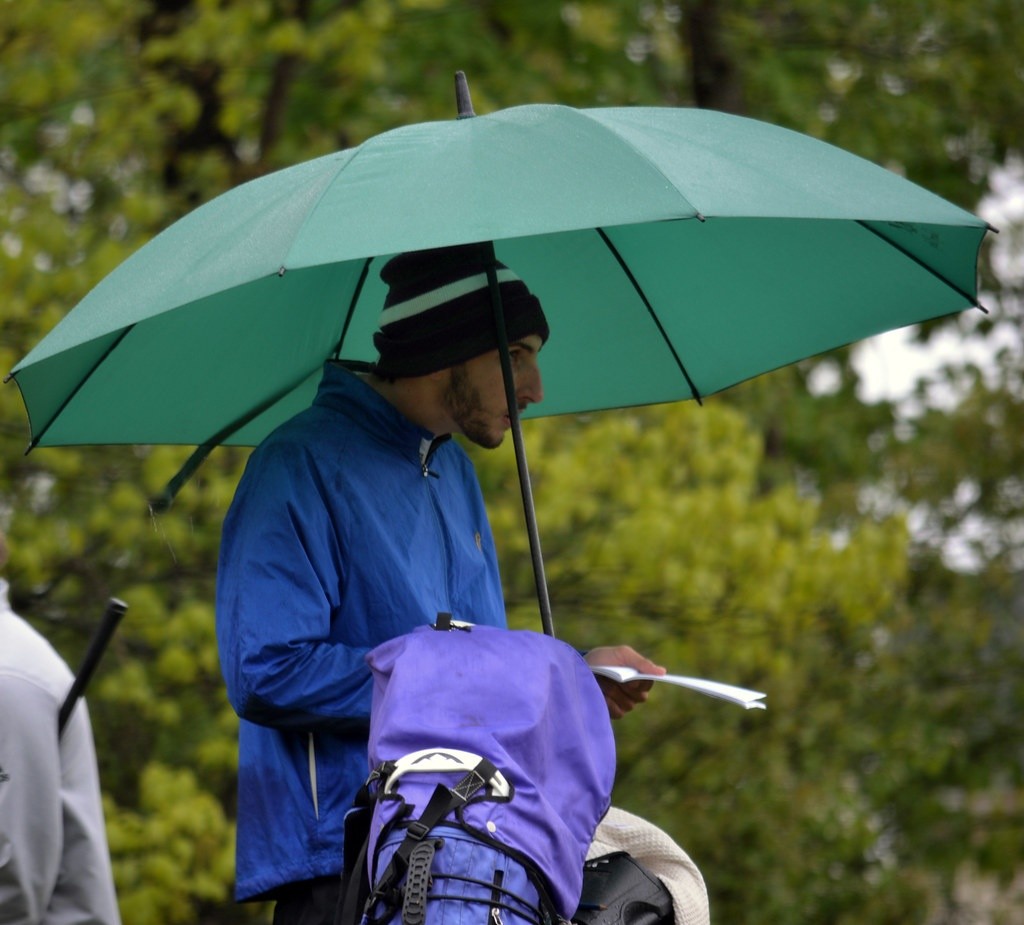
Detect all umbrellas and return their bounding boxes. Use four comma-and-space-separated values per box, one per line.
7, 70, 998, 635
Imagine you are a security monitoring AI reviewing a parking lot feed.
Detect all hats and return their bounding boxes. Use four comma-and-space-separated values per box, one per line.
372, 236, 549, 384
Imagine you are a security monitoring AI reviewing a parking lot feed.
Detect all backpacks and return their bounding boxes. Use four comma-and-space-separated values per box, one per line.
336, 613, 616, 925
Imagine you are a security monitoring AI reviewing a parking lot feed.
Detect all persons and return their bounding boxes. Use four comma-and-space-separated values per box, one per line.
0, 576, 123, 925
214, 237, 708, 924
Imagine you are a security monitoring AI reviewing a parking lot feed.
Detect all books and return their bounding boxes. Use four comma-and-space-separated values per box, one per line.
588, 663, 767, 714
